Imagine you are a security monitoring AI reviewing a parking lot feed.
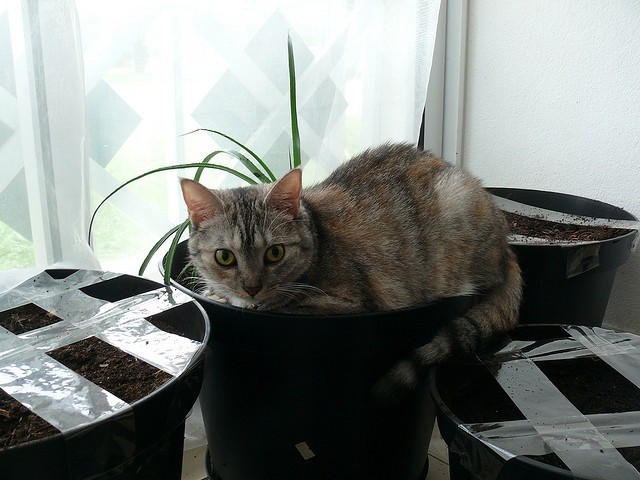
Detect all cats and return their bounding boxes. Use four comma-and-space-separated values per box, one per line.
181, 144, 525, 407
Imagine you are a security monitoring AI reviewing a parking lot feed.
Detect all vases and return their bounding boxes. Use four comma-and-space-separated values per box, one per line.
481, 187, 639, 328
0, 269, 210, 479
428, 322, 640, 479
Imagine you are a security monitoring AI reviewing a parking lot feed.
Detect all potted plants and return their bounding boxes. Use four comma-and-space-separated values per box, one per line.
88, 35, 475, 480
